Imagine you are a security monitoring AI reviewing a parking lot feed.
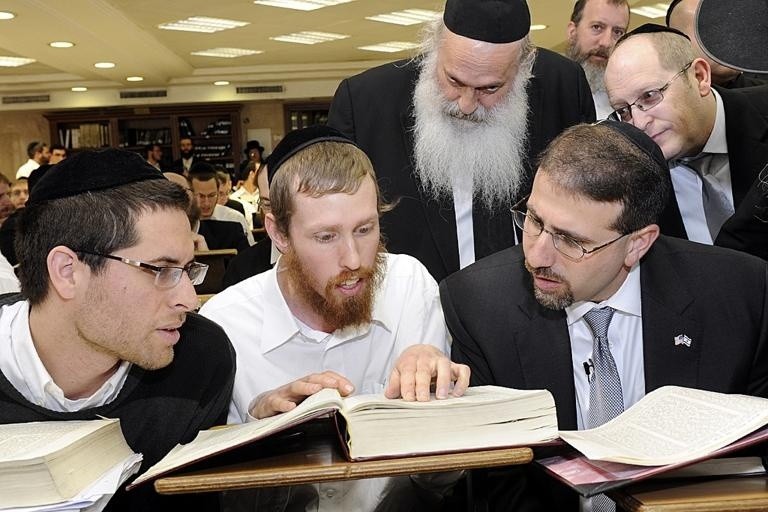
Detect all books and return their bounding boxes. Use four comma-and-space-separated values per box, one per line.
537, 385, 768, 499
0, 417, 145, 512
125, 383, 560, 492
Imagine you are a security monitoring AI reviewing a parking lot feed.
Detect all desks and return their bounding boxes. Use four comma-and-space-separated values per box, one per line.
189, 249, 237, 293
288, 151, 596, 423
153, 406, 533, 512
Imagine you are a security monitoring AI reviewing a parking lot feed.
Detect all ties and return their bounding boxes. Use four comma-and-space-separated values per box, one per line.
682, 155, 733, 243
583, 307, 625, 432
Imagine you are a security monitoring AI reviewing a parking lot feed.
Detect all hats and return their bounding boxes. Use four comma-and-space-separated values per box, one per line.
443, 0, 531, 44
25, 148, 168, 205
243, 140, 264, 154
615, 23, 691, 46
265, 127, 354, 189
601, 118, 667, 172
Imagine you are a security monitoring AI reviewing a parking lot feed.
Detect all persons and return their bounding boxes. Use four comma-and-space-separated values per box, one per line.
230, 160, 260, 207
15, 142, 52, 179
172, 134, 203, 179
602, 23, 767, 260
163, 171, 251, 251
0, 146, 237, 512
665, 1, 768, 89
0, 174, 14, 228
196, 125, 472, 512
239, 140, 265, 171
220, 163, 283, 291
144, 144, 169, 173
214, 165, 246, 217
438, 120, 768, 512
11, 176, 30, 209
28, 144, 68, 192
191, 165, 258, 247
548, 1, 630, 119
325, 1, 598, 286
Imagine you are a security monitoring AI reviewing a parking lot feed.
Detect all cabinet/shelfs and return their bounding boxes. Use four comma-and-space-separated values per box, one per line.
43, 101, 242, 187
281, 99, 334, 137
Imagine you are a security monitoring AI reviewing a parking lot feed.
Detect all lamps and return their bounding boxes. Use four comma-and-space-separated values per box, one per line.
125, 75, 144, 82
93, 60, 116, 69
48, 40, 76, 48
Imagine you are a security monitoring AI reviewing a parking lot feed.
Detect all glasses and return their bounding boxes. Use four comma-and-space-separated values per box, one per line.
608, 62, 692, 122
509, 194, 627, 261
77, 246, 210, 291
256, 197, 271, 210
